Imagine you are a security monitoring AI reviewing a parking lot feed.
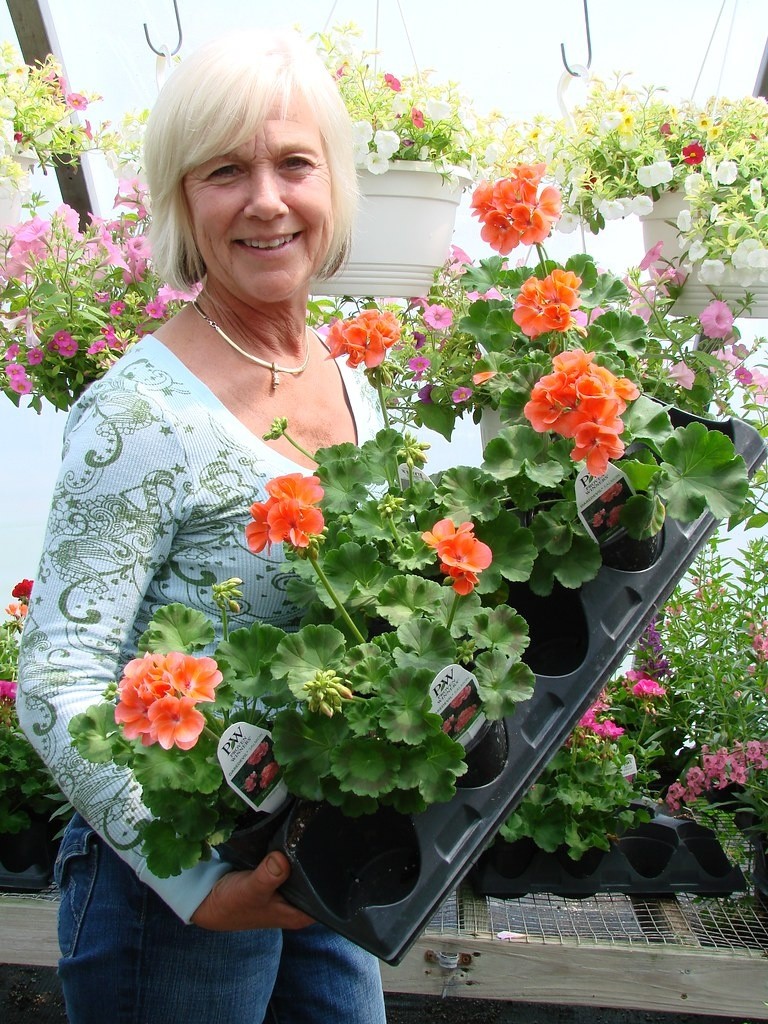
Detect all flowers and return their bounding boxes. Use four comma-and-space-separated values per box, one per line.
0, 18, 768, 878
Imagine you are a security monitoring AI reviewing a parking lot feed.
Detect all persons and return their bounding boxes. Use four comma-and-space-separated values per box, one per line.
17, 32, 397, 1023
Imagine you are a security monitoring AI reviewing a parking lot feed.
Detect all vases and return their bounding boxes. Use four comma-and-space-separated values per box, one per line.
227, 793, 293, 850
458, 717, 506, 789
599, 522, 664, 572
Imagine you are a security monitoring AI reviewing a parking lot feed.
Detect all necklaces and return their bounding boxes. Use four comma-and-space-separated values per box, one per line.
188, 298, 313, 388
